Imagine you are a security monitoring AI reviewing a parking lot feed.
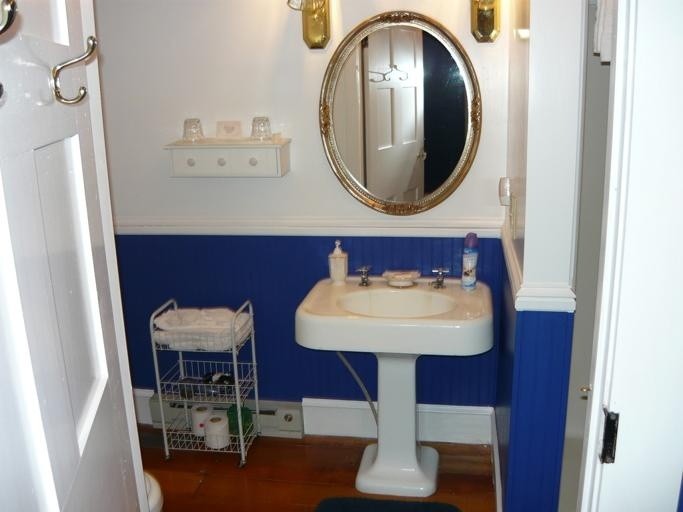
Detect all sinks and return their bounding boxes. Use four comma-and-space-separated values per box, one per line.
295, 274, 492, 356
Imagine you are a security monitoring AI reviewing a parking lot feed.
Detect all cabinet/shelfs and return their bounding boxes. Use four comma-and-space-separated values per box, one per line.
150, 298, 260, 468
164, 138, 292, 178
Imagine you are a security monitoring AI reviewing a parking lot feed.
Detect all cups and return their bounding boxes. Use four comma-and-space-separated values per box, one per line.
249, 116, 273, 143
181, 118, 205, 145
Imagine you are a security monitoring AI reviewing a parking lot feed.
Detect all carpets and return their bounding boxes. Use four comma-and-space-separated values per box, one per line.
314, 497, 462, 512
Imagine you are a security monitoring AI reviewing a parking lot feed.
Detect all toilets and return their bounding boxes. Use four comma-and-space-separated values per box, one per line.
143, 471, 164, 512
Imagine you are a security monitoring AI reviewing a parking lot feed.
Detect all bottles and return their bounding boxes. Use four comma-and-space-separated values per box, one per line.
461, 232, 480, 291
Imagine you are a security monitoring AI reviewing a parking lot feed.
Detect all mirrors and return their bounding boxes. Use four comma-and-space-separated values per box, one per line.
319, 10, 483, 216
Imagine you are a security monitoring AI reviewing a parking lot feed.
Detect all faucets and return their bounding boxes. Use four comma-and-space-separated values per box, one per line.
357, 267, 371, 288
428, 268, 448, 290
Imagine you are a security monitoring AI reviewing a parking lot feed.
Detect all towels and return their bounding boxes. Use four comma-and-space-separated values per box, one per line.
154, 307, 250, 351
